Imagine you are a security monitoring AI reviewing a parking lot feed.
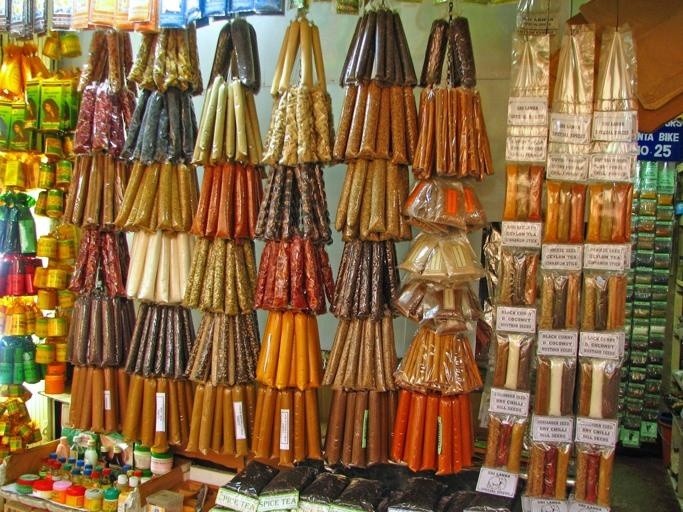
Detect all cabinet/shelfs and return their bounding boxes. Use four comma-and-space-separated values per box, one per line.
0, 433, 241, 512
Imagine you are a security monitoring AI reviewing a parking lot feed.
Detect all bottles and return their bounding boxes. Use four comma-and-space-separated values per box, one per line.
15, 474, 39, 493
52, 481, 71, 503
84, 465, 151, 493
83, 442, 98, 464
56, 437, 70, 459
103, 488, 119, 512
32, 481, 53, 499
84, 488, 103, 512
66, 486, 84, 507
38, 454, 82, 485
118, 492, 135, 512
150, 452, 173, 474
108, 446, 124, 469
70, 436, 83, 459
134, 443, 150, 469
